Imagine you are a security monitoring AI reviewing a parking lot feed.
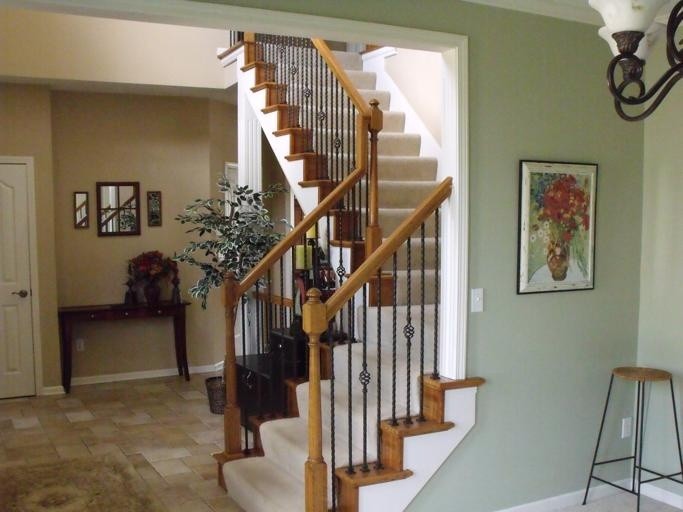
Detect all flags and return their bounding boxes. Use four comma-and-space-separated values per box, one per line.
143, 281, 161, 305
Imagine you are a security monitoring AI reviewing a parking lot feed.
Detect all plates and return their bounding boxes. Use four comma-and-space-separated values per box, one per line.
236, 350, 277, 432
270, 327, 347, 417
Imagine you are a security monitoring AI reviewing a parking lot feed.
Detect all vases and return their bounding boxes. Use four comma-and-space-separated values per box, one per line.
126, 249, 179, 289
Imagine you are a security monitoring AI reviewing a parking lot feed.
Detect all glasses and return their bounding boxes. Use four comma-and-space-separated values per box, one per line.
586, 0, 683, 125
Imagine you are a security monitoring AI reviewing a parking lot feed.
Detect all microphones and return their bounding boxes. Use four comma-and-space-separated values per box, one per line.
581, 365, 683, 512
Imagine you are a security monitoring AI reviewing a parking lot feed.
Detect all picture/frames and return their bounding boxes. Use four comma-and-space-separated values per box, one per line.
57, 300, 193, 388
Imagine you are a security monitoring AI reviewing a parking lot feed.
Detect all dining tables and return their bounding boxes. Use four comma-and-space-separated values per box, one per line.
170, 171, 295, 415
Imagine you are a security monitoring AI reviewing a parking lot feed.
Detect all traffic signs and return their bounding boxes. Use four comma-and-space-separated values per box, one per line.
620, 416, 633, 439
75, 335, 86, 352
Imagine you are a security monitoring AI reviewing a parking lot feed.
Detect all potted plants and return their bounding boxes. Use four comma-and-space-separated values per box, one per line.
516, 159, 599, 295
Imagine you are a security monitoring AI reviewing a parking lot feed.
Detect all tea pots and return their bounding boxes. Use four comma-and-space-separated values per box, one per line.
74, 192, 88, 229
147, 191, 163, 227
96, 182, 141, 236
